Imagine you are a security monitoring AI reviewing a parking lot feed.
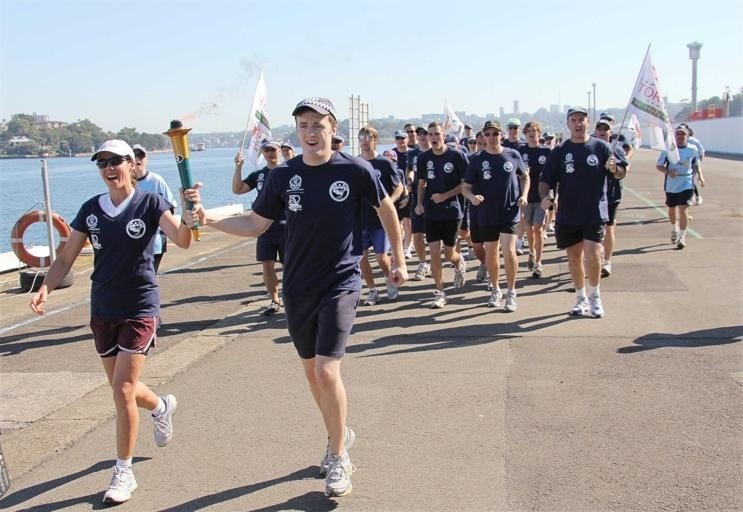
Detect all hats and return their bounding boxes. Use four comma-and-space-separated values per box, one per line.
331, 135, 344, 142
595, 112, 614, 134
675, 126, 689, 134
292, 97, 338, 122
132, 144, 146, 156
395, 118, 556, 143
567, 107, 588, 117
260, 138, 295, 152
383, 150, 397, 161
91, 139, 135, 162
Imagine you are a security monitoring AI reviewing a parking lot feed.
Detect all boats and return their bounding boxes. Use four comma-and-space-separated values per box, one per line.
191, 143, 204, 152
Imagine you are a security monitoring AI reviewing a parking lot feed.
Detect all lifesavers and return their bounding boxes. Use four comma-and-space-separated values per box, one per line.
10, 210, 71, 267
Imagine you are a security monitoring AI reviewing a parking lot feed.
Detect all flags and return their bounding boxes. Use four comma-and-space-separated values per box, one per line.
613, 112, 641, 153
633, 53, 681, 166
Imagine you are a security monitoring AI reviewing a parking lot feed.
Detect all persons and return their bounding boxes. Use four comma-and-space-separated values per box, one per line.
655, 125, 705, 247
29, 139, 204, 505
233, 105, 631, 318
688, 128, 694, 136
129, 144, 177, 330
179, 99, 408, 495
677, 123, 702, 206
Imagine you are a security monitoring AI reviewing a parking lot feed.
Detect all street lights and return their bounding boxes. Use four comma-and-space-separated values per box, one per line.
586, 90, 592, 121
684, 39, 704, 118
591, 83, 597, 127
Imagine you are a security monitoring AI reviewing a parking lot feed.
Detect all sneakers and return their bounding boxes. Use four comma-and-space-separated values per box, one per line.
542, 224, 555, 239
364, 241, 542, 312
677, 237, 685, 247
571, 294, 589, 312
151, 395, 177, 447
103, 466, 137, 503
601, 265, 611, 275
671, 231, 678, 244
267, 301, 281, 311
688, 198, 695, 206
590, 296, 605, 317
696, 196, 703, 205
321, 426, 355, 497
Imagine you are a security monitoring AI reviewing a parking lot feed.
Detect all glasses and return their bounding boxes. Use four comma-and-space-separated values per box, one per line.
96, 156, 129, 168
135, 152, 145, 159
359, 135, 373, 141
